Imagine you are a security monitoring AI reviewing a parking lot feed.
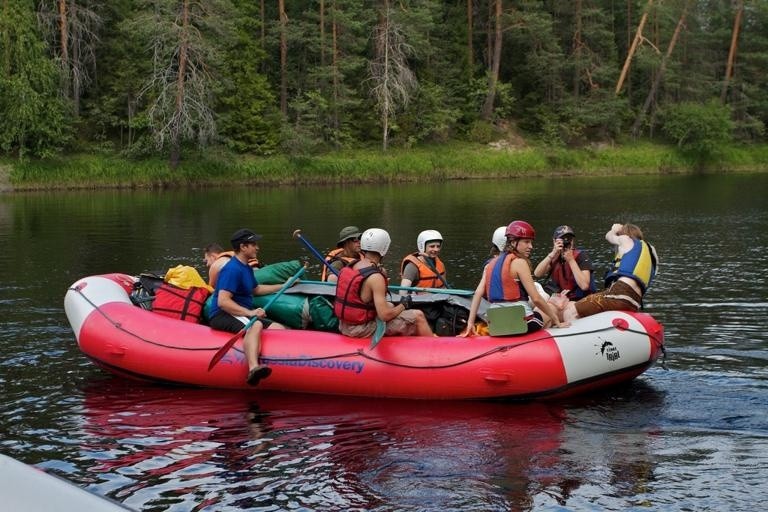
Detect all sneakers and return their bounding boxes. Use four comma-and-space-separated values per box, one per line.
247, 365, 271, 385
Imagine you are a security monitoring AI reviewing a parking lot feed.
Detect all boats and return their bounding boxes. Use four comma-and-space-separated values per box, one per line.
63, 272, 664, 401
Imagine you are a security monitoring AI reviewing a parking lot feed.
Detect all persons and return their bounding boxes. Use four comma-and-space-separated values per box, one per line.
335, 225, 442, 338
485, 226, 554, 309
203, 241, 261, 292
533, 224, 596, 301
208, 227, 301, 387
462, 218, 572, 338
395, 230, 470, 337
562, 223, 659, 322
322, 225, 391, 285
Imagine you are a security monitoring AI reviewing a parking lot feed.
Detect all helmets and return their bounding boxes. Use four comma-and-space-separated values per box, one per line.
492, 221, 535, 252
360, 228, 391, 256
417, 230, 443, 253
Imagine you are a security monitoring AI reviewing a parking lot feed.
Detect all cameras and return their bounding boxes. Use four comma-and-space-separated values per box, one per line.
563, 240, 571, 249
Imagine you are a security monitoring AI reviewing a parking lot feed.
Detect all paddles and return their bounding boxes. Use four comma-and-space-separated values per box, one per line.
297, 279, 474, 297
208, 261, 309, 371
369, 315, 386, 348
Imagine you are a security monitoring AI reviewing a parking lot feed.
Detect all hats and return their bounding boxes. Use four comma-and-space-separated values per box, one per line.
231, 229, 263, 249
337, 226, 362, 248
553, 226, 575, 239
